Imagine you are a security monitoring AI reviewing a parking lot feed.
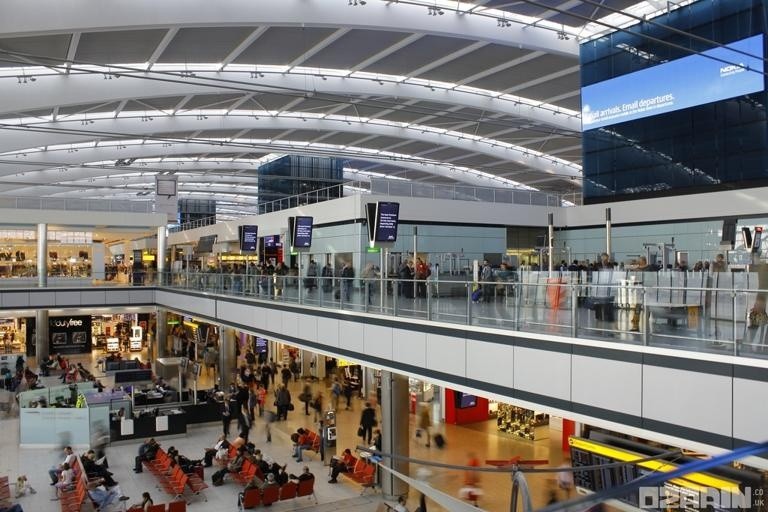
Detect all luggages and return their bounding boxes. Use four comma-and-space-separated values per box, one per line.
435, 434, 445, 447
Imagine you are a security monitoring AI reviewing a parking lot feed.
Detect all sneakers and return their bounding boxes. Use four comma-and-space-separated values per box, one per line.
292, 454, 303, 462
50, 482, 56, 485
119, 496, 129, 501
328, 479, 337, 483
204, 463, 212, 467
133, 468, 143, 473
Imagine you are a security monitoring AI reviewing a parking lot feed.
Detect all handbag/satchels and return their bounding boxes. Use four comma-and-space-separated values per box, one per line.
287, 403, 293, 410
212, 471, 222, 484
357, 425, 364, 436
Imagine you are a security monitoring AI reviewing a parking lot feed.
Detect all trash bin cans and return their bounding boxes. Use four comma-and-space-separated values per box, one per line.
324, 424, 337, 447
132, 262, 144, 285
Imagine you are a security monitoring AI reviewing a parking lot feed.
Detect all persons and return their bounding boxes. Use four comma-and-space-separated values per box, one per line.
0, 348, 433, 511
559, 457, 573, 500
127, 254, 725, 304
546, 490, 559, 506
